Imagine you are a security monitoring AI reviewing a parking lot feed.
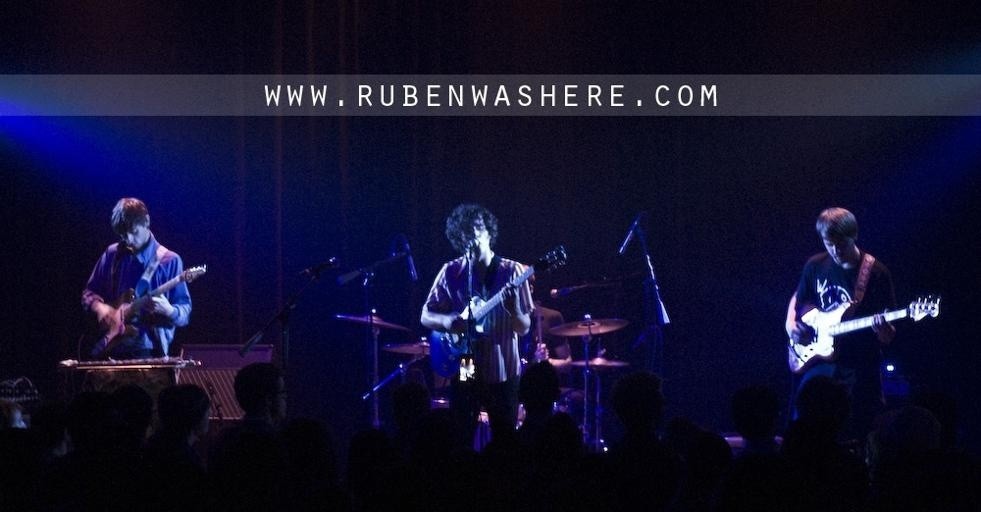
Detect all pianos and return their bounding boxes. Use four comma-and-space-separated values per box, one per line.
55, 356, 201, 386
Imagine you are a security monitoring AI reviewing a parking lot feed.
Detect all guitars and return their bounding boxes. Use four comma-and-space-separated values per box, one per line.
74, 267, 209, 360
787, 296, 940, 374
430, 246, 567, 379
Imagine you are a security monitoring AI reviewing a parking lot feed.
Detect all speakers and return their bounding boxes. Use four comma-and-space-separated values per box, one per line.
177, 346, 272, 422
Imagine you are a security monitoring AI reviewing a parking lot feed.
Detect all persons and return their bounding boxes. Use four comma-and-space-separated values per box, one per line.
79, 196, 192, 355
418, 201, 535, 428
785, 205, 897, 422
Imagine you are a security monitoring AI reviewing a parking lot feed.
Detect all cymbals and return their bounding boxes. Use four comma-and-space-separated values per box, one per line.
570, 358, 629, 367
385, 343, 432, 357
549, 318, 629, 336
334, 313, 408, 333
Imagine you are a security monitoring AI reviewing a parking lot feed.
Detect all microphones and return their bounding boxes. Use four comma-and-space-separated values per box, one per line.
300, 257, 336, 275
404, 242, 420, 281
617, 215, 646, 254
465, 238, 479, 250
